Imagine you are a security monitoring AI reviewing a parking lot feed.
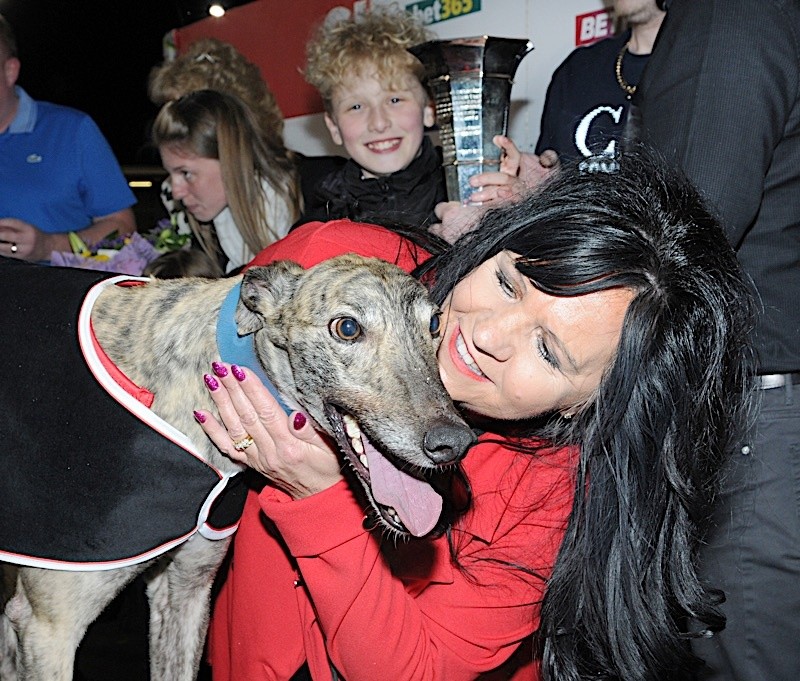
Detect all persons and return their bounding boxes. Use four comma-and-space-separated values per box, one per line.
210, 134, 761, 681
428, 0, 800, 681
141, 0, 672, 280
0, 14, 138, 262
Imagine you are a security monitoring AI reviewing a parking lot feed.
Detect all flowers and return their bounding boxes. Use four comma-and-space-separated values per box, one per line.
51, 212, 192, 278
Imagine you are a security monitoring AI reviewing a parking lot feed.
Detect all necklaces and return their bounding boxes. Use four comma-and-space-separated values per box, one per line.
616, 38, 636, 100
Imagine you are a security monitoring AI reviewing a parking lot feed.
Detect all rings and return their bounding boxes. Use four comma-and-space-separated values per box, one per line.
232, 435, 253, 451
11, 243, 17, 253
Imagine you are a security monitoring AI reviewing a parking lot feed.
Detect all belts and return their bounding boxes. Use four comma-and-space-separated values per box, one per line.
747, 373, 800, 390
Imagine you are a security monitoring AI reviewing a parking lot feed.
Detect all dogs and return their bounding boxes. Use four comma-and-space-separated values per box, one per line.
0, 250, 477, 681
